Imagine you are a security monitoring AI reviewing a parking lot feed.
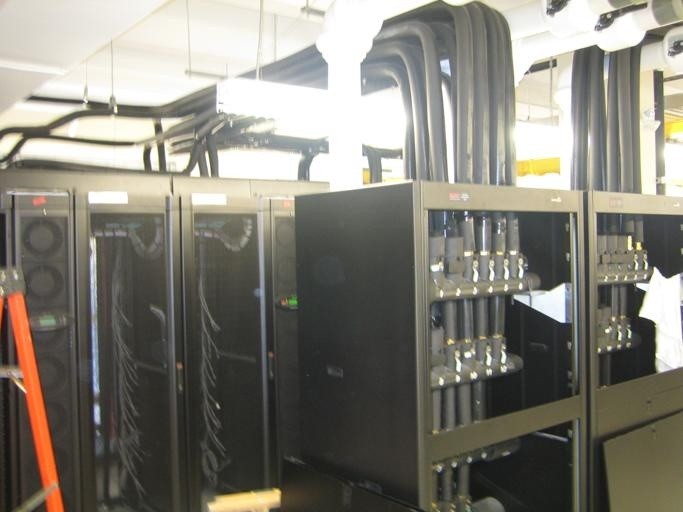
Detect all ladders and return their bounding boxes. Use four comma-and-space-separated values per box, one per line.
0, 265, 64, 512
